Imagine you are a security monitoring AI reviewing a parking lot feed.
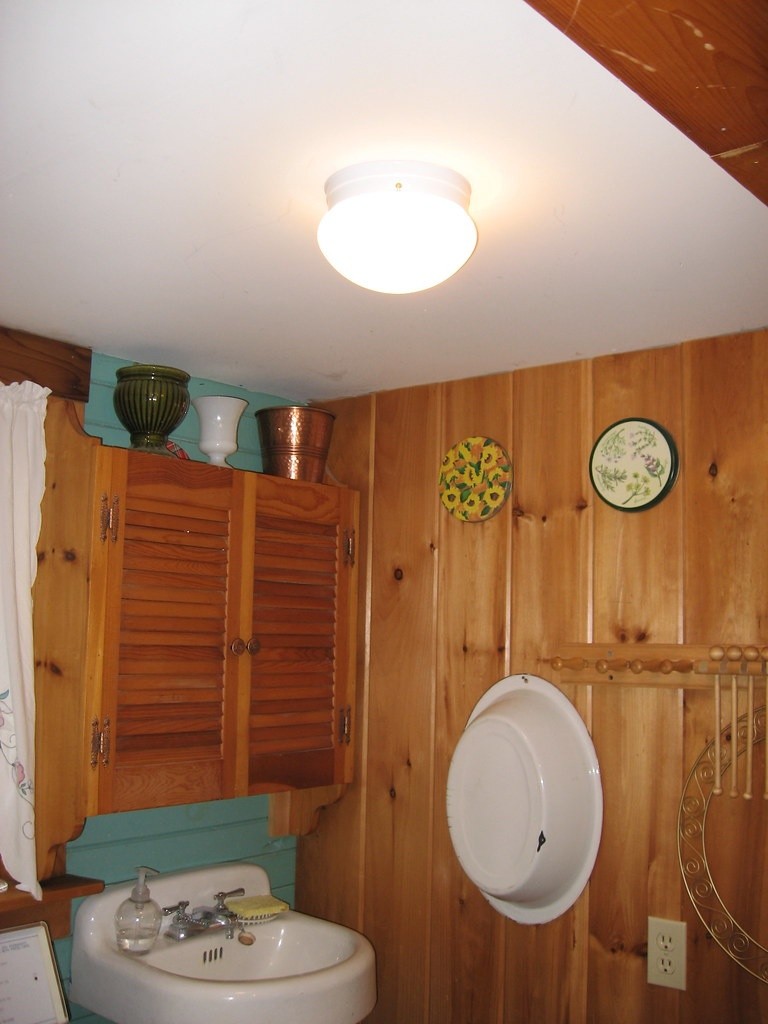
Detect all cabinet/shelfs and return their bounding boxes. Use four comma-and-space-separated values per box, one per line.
31, 396, 361, 882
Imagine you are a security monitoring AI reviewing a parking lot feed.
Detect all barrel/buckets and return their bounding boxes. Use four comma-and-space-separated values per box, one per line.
254, 406, 336, 485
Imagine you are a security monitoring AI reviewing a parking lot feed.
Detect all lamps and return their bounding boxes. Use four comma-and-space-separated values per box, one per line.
316, 162, 478, 294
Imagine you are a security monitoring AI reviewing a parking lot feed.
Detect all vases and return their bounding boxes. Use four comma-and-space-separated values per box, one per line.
191, 396, 248, 469
254, 405, 337, 483
113, 364, 191, 456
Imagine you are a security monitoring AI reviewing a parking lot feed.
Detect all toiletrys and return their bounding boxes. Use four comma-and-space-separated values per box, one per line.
115, 866, 163, 956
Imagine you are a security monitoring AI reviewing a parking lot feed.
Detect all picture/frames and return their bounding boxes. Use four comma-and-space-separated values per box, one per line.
589, 417, 679, 514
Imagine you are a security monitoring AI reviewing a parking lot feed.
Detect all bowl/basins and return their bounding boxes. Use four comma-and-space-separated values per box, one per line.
447, 673, 604, 924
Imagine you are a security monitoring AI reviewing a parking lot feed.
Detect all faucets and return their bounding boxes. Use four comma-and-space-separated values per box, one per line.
161, 887, 245, 942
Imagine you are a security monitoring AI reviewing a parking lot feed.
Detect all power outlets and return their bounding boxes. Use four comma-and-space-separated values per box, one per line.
647, 916, 687, 991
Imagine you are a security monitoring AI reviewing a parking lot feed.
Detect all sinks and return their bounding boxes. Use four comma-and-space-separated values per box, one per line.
67, 860, 377, 1023
